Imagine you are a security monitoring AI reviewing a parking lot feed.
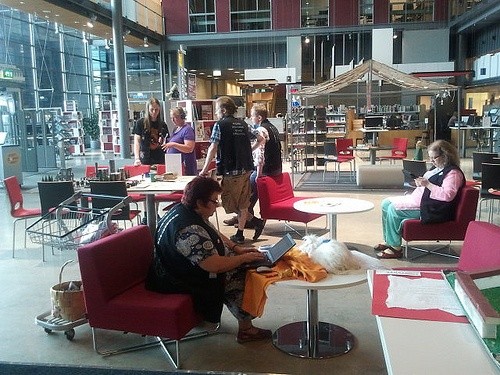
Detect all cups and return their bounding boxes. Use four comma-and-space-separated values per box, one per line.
149, 173, 157, 182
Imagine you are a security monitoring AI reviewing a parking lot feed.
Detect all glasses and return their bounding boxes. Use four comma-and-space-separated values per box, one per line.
206, 197, 219, 205
427, 153, 441, 159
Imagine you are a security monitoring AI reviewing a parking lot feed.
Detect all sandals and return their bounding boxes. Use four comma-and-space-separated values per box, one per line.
374, 242, 404, 258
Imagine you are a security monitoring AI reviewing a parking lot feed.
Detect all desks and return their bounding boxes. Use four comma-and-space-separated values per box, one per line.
449, 125, 499, 156
355, 144, 393, 166
272, 250, 500, 375
125, 172, 193, 227
292, 197, 376, 240
360, 129, 388, 143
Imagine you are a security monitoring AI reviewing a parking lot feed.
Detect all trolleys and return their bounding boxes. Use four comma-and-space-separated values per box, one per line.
26, 191, 144, 341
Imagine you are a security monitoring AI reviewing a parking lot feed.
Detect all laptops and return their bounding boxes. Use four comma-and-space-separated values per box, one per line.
242, 233, 296, 268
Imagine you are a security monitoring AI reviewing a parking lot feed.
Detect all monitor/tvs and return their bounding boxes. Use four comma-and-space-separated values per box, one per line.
462, 115, 469, 122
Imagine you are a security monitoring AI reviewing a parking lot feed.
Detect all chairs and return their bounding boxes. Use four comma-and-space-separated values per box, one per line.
401, 151, 500, 271
380, 137, 408, 166
4, 165, 223, 367
255, 172, 324, 238
320, 140, 355, 184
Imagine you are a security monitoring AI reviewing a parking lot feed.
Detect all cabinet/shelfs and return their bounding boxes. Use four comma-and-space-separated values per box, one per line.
327, 102, 419, 132
177, 99, 219, 157
57, 110, 133, 157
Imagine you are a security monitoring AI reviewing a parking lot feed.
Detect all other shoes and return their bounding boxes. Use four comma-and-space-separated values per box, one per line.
251, 217, 266, 241
229, 234, 245, 245
223, 216, 239, 225
237, 326, 276, 344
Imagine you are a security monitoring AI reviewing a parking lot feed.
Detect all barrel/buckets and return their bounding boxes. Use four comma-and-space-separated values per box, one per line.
50, 260, 86, 321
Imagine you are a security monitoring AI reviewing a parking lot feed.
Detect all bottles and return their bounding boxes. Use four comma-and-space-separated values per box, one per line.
41, 161, 126, 188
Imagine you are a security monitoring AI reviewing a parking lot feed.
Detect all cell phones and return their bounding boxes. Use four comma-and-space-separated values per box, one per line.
409, 172, 419, 179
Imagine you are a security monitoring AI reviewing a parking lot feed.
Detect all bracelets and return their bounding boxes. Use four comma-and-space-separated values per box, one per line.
232, 244, 239, 253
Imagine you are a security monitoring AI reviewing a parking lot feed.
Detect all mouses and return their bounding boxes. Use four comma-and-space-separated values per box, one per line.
256, 266, 272, 273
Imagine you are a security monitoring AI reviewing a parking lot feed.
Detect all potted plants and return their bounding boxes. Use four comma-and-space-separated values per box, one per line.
83, 115, 99, 149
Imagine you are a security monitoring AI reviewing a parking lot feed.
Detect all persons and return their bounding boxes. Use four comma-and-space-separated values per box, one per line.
161, 107, 198, 176
447, 112, 461, 127
155, 176, 273, 343
132, 98, 170, 226
374, 140, 466, 258
198, 96, 282, 244
